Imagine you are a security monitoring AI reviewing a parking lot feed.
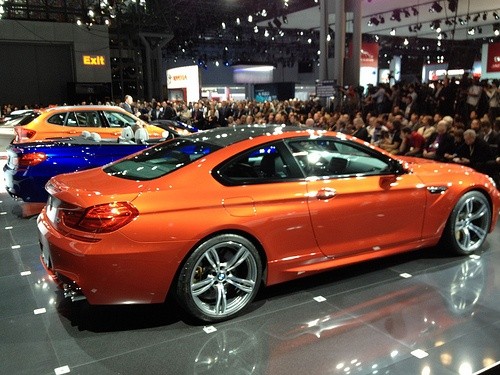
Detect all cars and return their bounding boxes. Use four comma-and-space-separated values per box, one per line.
5, 105, 194, 143
35, 126, 499, 323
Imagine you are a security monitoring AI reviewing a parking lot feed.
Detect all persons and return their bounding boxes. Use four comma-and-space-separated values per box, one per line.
0, 72, 500, 194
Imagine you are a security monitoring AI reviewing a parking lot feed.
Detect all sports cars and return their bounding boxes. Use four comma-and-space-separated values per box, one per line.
2, 119, 281, 203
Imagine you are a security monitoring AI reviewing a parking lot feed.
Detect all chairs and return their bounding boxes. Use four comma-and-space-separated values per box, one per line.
261, 153, 287, 179
82, 125, 150, 143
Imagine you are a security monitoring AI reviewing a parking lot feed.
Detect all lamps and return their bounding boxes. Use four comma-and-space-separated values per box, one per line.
367, 0, 499, 33
